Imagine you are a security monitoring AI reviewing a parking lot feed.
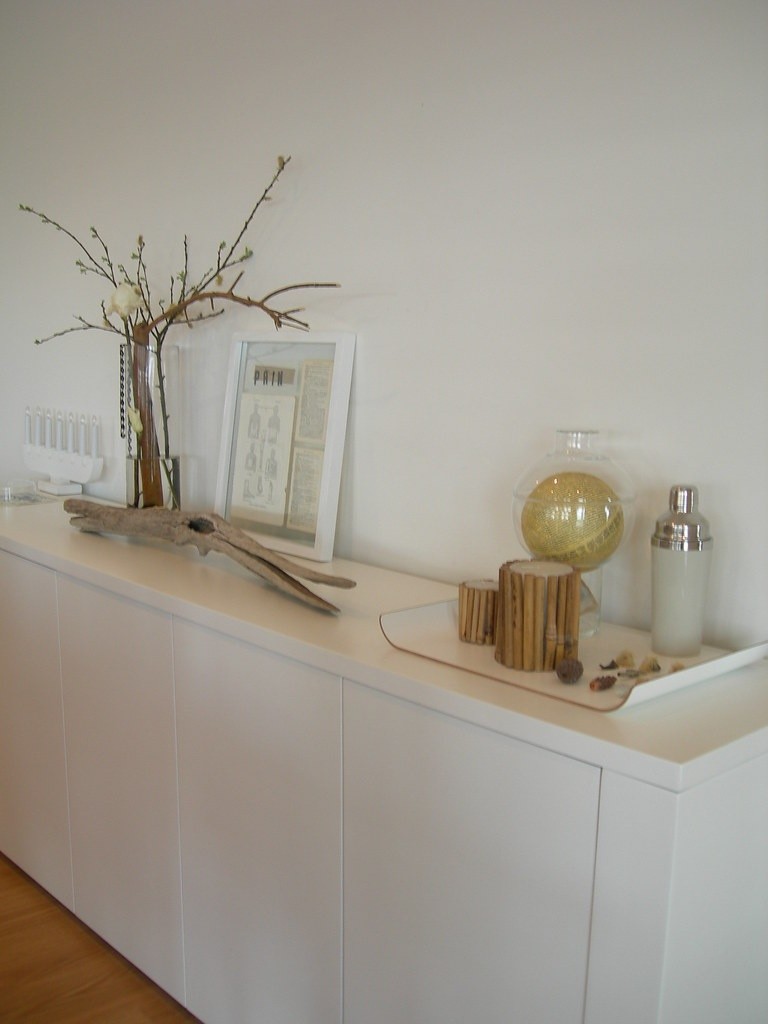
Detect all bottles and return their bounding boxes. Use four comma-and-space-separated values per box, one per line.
650, 485, 713, 655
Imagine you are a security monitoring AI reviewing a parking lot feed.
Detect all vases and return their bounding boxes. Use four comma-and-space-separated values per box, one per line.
124, 345, 180, 507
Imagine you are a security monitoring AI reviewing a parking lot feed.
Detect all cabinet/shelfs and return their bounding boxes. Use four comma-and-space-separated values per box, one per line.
0, 494, 768, 1024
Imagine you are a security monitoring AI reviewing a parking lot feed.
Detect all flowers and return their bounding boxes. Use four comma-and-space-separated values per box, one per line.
20, 156, 341, 507
